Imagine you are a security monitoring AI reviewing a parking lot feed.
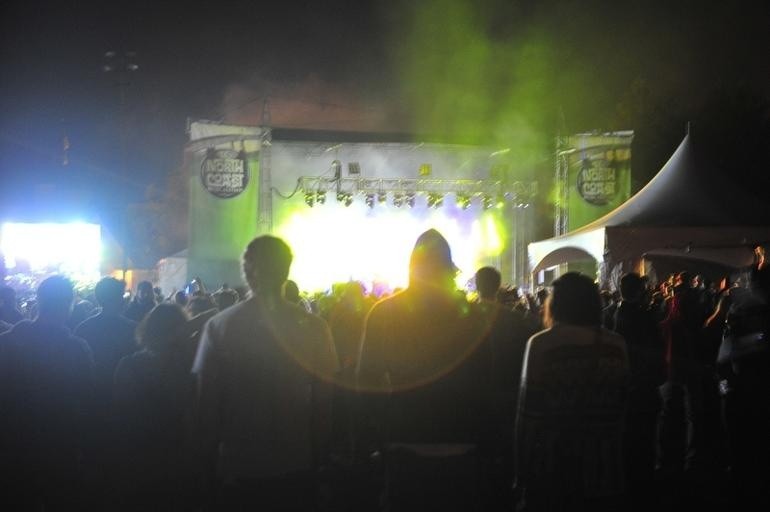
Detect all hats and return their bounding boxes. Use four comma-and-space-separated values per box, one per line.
408, 228, 458, 281
617, 274, 649, 297
